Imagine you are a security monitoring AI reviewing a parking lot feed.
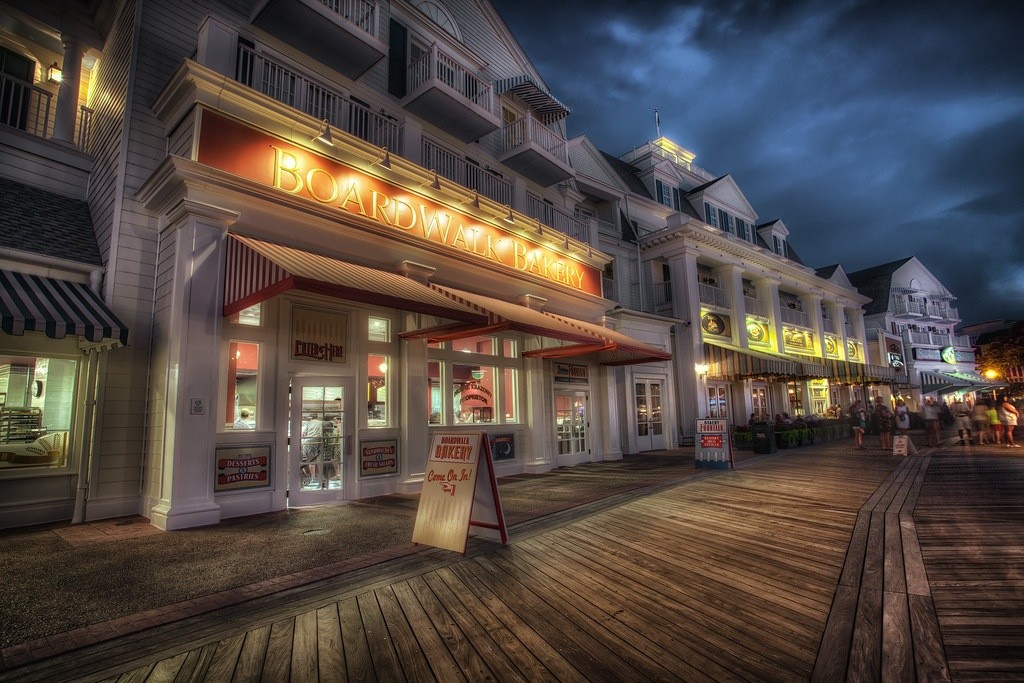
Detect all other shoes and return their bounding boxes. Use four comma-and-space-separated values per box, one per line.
1006, 444, 1021, 448
937, 443, 944, 448
881, 446, 893, 450
969, 438, 976, 445
928, 441, 934, 447
854, 445, 866, 451
961, 439, 966, 445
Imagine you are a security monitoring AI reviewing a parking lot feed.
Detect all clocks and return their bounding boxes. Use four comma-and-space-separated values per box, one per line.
31, 380, 42, 397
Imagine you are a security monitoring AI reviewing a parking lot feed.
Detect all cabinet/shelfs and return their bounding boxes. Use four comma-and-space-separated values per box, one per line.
0, 407, 42, 444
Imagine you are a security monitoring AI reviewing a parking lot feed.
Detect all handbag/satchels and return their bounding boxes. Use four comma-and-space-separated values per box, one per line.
859, 426, 865, 433
899, 414, 906, 421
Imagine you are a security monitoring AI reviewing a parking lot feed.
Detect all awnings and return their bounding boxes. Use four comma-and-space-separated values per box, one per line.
704, 341, 908, 386
919, 370, 1009, 396
0, 269, 128, 354
222, 234, 672, 368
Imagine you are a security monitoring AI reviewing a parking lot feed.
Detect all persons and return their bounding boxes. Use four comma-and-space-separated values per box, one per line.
232, 409, 251, 429
749, 393, 1020, 450
302, 413, 342, 482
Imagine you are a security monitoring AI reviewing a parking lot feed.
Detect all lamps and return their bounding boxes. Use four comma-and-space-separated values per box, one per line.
460, 189, 479, 209
419, 169, 441, 191
574, 242, 592, 259
523, 218, 542, 236
551, 232, 569, 250
492, 204, 514, 224
311, 119, 334, 147
371, 147, 391, 172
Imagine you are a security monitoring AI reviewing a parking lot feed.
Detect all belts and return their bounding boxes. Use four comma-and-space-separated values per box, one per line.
955, 415, 968, 418
